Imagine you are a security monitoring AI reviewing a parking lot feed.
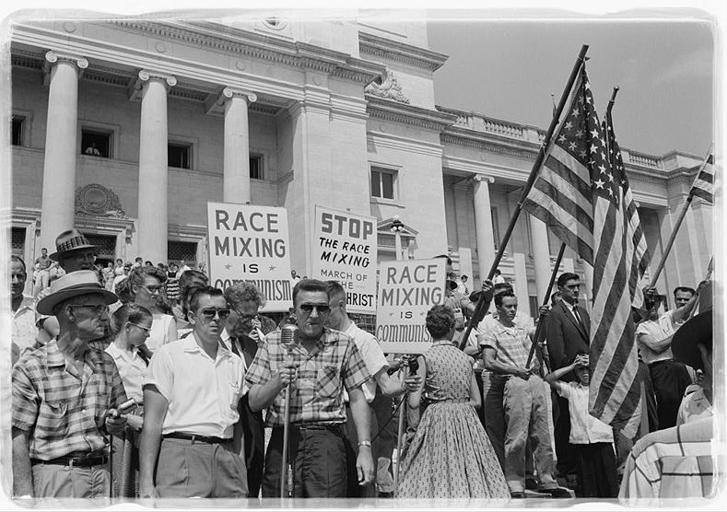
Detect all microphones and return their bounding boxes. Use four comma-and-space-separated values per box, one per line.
408, 357, 419, 376
280, 323, 301, 351
117, 397, 139, 415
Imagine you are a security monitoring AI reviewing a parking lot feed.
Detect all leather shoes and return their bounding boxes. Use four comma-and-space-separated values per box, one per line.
511, 491, 526, 498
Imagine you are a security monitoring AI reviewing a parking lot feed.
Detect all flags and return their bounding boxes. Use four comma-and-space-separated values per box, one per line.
519, 56, 653, 439
689, 145, 715, 206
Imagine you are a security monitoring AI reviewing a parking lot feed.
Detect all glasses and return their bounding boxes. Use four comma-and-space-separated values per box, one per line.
194, 307, 231, 320
72, 304, 110, 317
75, 254, 97, 265
297, 303, 329, 313
231, 305, 259, 324
137, 284, 161, 291
446, 272, 456, 281
129, 322, 151, 332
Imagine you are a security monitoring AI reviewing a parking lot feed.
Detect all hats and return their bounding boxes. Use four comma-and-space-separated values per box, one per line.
671, 284, 712, 370
36, 270, 118, 316
49, 230, 101, 261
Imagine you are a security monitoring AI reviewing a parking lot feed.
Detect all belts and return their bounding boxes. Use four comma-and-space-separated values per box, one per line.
163, 432, 234, 444
297, 425, 325, 430
30, 457, 108, 467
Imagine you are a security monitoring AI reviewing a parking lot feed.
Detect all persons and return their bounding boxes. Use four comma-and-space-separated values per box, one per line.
10, 229, 713, 501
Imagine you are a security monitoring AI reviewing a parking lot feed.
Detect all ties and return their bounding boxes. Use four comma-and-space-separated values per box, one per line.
573, 305, 584, 331
